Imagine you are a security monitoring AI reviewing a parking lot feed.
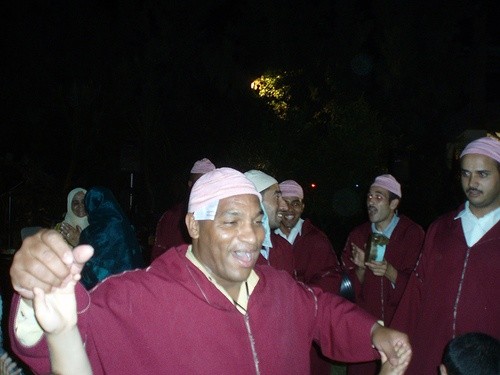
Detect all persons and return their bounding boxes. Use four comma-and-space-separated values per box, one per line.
378, 331, 500, 375
8, 166, 413, 375
32, 264, 96, 375
151, 171, 205, 261
387, 137, 500, 375
271, 179, 344, 295
339, 173, 426, 374
243, 168, 297, 282
51, 187, 91, 249
78, 182, 148, 291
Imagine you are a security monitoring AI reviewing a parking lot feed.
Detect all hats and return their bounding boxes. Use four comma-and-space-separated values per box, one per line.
188, 168, 273, 261
371, 174, 402, 198
244, 170, 278, 192
279, 180, 303, 200
190, 158, 215, 174
460, 137, 500, 163
